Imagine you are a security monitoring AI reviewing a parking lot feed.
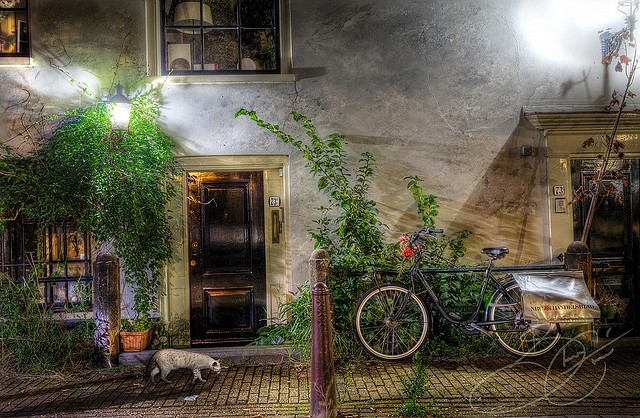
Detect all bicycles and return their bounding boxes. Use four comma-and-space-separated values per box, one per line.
355, 226, 564, 362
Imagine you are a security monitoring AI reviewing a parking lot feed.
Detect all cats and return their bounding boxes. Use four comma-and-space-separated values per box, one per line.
145, 348, 224, 387
